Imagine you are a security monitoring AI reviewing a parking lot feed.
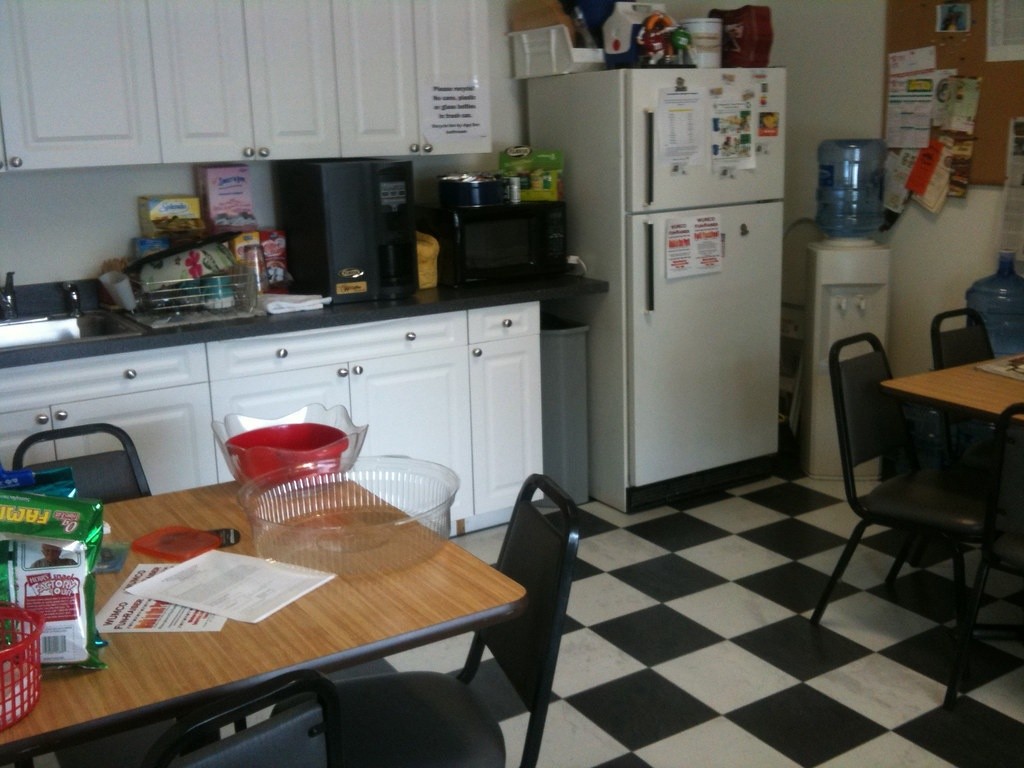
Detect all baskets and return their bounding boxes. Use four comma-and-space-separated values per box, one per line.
0, 606, 44, 732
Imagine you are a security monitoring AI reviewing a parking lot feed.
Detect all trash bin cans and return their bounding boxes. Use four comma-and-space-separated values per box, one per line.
539, 315, 593, 508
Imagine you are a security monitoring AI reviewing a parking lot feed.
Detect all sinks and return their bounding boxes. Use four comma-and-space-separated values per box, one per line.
0, 305, 151, 354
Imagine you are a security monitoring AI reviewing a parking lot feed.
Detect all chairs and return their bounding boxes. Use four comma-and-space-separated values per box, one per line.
810, 332, 987, 642
54, 669, 343, 768
942, 402, 1024, 710
272, 474, 580, 768
909, 309, 998, 566
13, 423, 152, 504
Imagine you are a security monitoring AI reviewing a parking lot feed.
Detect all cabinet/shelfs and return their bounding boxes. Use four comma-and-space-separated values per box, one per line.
1, 299, 543, 539
0, 0, 493, 173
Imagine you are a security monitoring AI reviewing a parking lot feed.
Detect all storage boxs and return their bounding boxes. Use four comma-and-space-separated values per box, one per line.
138, 195, 201, 239
229, 232, 264, 295
507, 24, 607, 80
195, 165, 258, 237
258, 229, 289, 294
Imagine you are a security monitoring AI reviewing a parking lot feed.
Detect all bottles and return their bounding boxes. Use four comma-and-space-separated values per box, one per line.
817, 138, 884, 238
963, 248, 1024, 365
244, 244, 268, 315
509, 177, 522, 202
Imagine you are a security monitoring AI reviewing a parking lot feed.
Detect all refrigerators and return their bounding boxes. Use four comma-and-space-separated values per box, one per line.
527, 66, 786, 511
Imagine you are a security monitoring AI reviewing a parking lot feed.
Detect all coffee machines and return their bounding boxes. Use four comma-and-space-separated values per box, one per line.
271, 160, 420, 303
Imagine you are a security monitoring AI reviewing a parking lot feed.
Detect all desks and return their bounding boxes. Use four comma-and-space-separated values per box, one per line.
880, 355, 1024, 639
0, 480, 529, 768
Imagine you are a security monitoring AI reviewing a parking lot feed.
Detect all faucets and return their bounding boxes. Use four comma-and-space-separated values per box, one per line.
2, 270, 18, 325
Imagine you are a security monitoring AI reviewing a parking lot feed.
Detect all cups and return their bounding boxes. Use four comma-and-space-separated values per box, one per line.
150, 274, 235, 314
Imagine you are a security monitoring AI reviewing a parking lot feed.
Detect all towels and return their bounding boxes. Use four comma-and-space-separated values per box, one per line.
260, 292, 324, 315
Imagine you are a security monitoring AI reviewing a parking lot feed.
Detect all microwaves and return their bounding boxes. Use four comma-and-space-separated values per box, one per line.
421, 199, 565, 290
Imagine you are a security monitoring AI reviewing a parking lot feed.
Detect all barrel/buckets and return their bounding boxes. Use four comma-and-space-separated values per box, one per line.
678, 18, 722, 70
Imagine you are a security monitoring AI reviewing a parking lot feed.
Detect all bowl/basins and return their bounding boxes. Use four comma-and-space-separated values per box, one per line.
440, 172, 503, 209
229, 426, 348, 488
211, 402, 369, 489
